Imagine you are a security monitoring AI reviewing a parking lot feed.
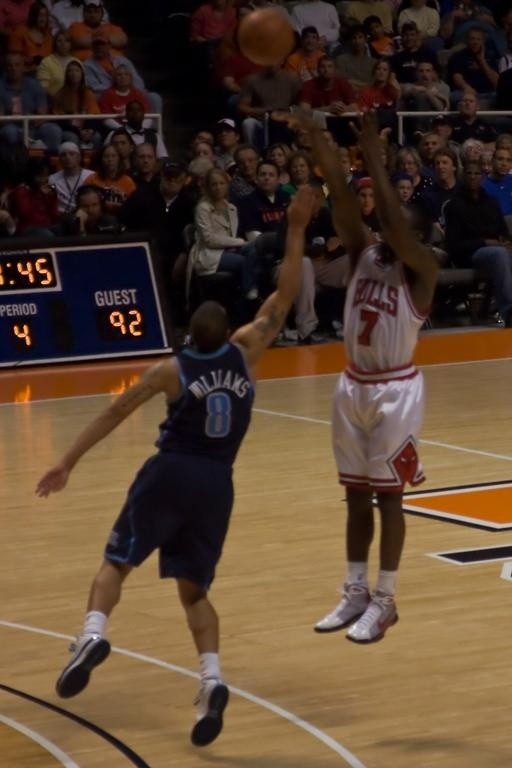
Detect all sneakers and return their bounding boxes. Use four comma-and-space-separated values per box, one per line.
56, 635, 110, 697
314, 582, 397, 643
191, 677, 229, 746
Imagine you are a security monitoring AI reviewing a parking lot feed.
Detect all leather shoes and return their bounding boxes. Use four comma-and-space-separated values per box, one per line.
297, 333, 325, 344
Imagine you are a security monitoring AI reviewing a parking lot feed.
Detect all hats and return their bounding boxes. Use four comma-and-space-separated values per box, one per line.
164, 155, 187, 175
356, 177, 373, 190
59, 142, 78, 153
217, 118, 235, 130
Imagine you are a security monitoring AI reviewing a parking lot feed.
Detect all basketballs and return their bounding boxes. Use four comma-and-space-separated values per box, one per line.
239, 10, 293, 64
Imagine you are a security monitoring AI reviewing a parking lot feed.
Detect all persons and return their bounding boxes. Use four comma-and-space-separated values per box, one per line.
35, 184, 319, 750
284, 102, 442, 645
0, 1, 511, 348
